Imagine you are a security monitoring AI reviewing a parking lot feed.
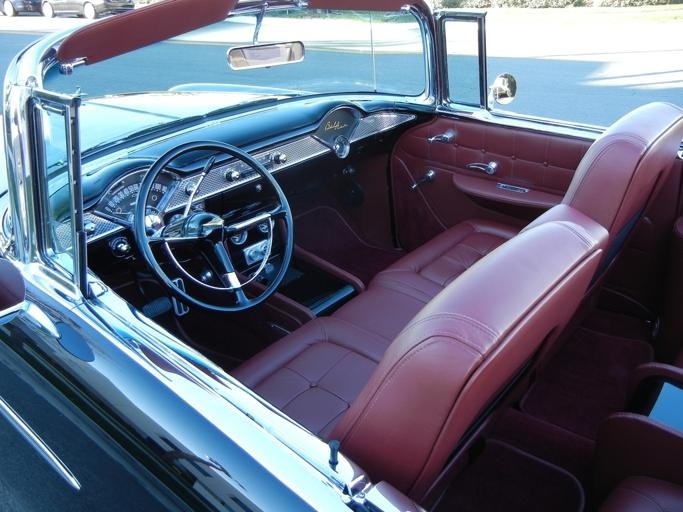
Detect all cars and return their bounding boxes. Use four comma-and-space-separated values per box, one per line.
0, 0, 135, 19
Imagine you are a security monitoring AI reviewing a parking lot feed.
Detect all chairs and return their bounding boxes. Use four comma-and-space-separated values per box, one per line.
227, 198, 607, 512
369, 103, 681, 408
601, 348, 682, 512
